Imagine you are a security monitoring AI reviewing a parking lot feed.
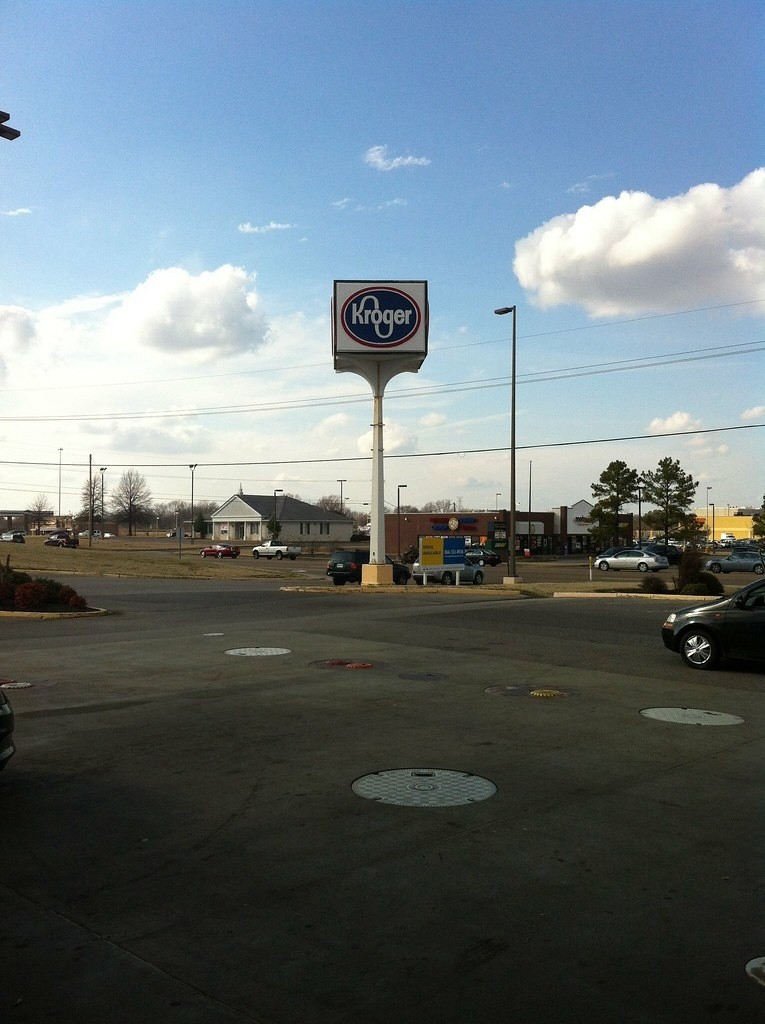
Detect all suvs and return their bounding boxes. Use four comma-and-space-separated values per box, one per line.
1, 530, 27, 538
326, 548, 411, 586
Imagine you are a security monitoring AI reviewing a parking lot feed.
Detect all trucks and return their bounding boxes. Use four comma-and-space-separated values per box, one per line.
721, 533, 736, 540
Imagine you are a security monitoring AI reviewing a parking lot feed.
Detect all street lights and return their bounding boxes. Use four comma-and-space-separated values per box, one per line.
397, 485, 407, 555
58, 448, 63, 518
100, 468, 107, 540
727, 504, 729, 516
709, 504, 715, 554
175, 511, 179, 529
528, 461, 532, 549
496, 494, 501, 510
190, 464, 198, 538
337, 480, 346, 510
494, 305, 519, 577
273, 489, 283, 540
344, 498, 349, 515
707, 487, 712, 546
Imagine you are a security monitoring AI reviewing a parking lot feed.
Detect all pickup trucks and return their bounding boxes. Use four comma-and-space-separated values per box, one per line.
252, 540, 302, 560
44, 534, 79, 549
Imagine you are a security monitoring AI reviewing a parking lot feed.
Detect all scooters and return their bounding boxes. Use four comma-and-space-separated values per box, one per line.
401, 552, 418, 565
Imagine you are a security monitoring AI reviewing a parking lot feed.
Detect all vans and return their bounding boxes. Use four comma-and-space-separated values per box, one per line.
78, 530, 101, 539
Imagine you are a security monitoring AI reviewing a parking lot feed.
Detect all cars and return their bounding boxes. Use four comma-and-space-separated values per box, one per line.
104, 533, 115, 538
48, 532, 68, 535
199, 543, 240, 559
170, 532, 188, 537
705, 545, 765, 575
593, 547, 669, 572
685, 540, 720, 549
642, 545, 677, 553
662, 578, 765, 669
720, 539, 759, 548
0, 689, 16, 771
636, 542, 655, 550
412, 557, 486, 585
3, 534, 15, 542
465, 549, 502, 567
